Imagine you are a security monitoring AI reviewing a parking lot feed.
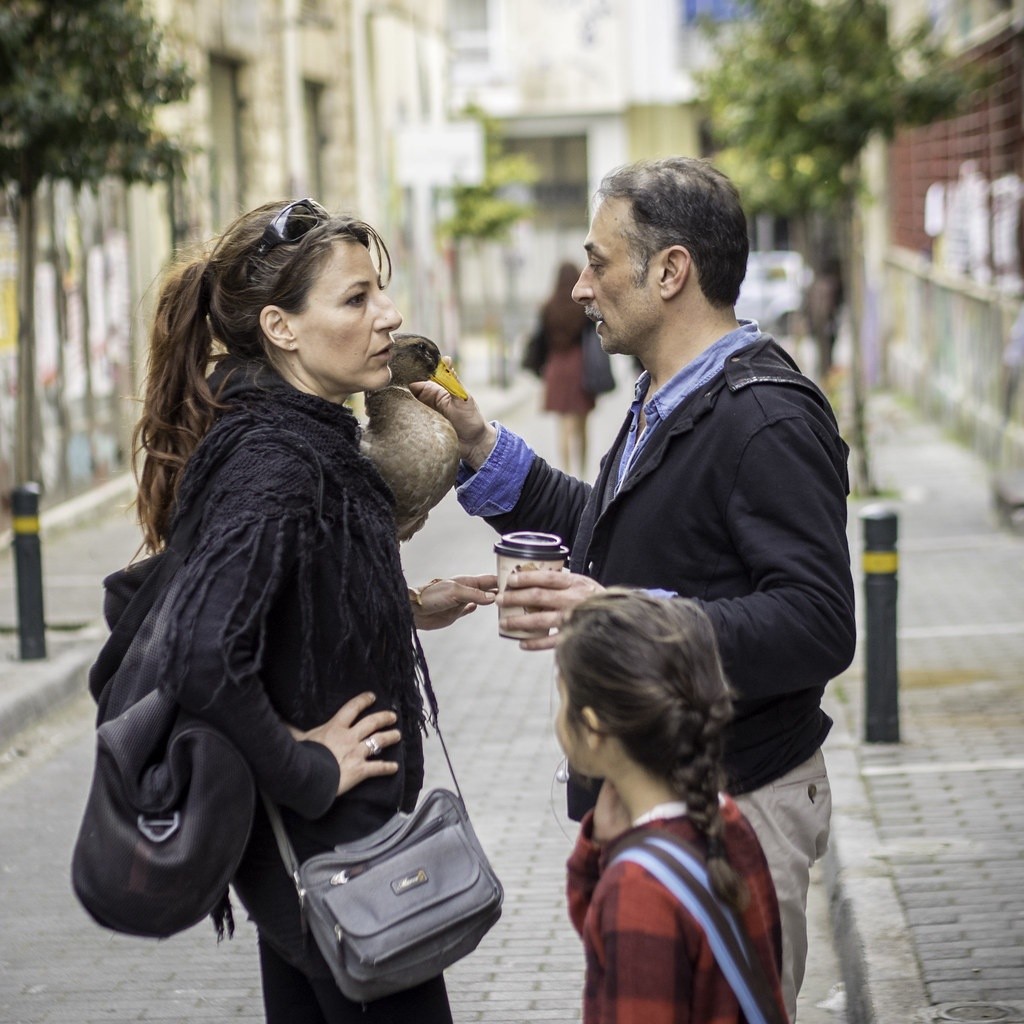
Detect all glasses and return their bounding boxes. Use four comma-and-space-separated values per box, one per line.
244, 197, 331, 280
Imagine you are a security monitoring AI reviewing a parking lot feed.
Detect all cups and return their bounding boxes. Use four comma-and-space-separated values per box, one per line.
493, 531, 571, 642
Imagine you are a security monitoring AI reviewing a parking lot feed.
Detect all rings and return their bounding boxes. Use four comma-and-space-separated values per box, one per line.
364, 737, 381, 755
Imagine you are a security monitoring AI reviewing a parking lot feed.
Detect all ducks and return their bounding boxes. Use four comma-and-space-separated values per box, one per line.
348, 331, 470, 542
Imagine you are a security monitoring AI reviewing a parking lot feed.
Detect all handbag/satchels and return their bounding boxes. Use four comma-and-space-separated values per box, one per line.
71, 552, 257, 939
577, 324, 617, 394
295, 789, 503, 1000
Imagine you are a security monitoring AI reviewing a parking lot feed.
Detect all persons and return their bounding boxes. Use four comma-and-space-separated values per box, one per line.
132, 199, 498, 1023
553, 588, 787, 1024
409, 156, 856, 1024
544, 263, 597, 479
